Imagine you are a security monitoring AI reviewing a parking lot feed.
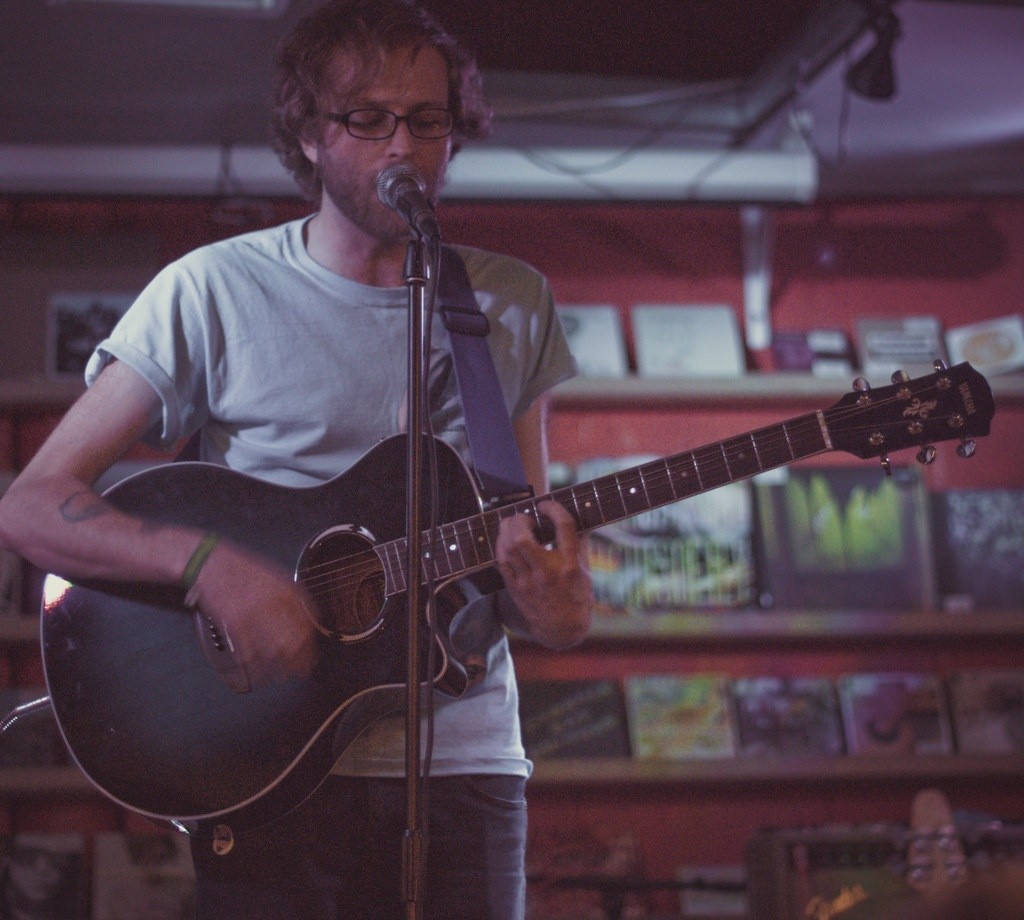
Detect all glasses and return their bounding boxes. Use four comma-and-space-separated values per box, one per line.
311, 107, 453, 141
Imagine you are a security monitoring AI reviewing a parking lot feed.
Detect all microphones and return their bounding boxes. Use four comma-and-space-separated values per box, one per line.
374, 159, 440, 244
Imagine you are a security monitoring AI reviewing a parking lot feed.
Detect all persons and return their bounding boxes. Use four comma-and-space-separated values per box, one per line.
0, 0, 596, 920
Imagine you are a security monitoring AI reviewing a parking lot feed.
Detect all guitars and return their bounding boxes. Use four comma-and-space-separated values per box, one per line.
42, 354, 1000, 827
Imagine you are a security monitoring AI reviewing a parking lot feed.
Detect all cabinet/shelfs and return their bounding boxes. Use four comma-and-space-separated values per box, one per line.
0, 371, 1024, 796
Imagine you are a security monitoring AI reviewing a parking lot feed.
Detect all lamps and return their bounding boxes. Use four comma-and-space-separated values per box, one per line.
844, 10, 906, 103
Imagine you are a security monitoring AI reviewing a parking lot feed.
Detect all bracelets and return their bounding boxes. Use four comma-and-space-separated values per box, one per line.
177, 531, 221, 591
